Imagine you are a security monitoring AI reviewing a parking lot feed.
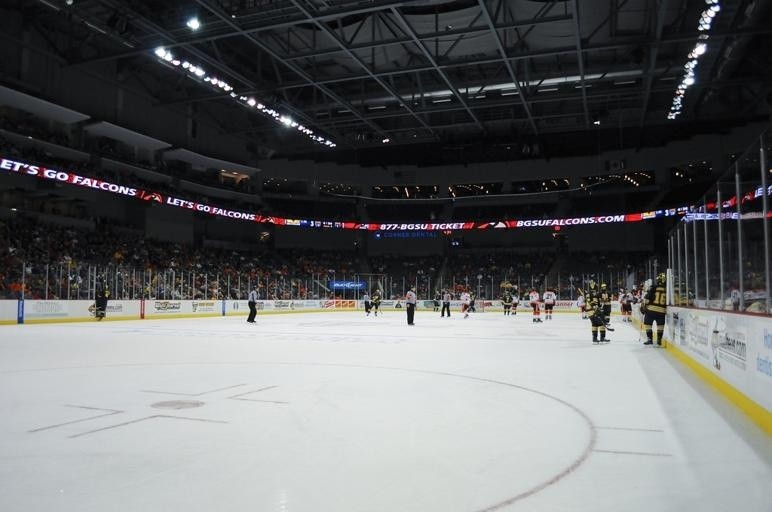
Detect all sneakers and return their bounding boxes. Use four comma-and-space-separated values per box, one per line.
643, 340, 653, 345
600, 338, 610, 342
593, 339, 600, 342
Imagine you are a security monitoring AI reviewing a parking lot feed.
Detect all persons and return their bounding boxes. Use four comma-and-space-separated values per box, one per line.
597, 283, 613, 326
502, 290, 510, 316
529, 288, 543, 322
1, 215, 770, 314
585, 280, 611, 345
576, 292, 587, 320
639, 271, 669, 346
246, 286, 260, 324
620, 290, 637, 322
543, 287, 557, 320
405, 287, 417, 325
441, 290, 451, 317
460, 290, 471, 319
512, 296, 520, 314
0, 107, 291, 215
367, 288, 383, 316
95, 285, 110, 320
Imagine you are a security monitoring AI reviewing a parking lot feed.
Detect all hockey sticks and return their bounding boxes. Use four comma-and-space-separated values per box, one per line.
639, 314, 644, 342
577, 286, 616, 332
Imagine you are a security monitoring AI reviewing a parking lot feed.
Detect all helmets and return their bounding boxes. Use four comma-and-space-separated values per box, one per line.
601, 283, 607, 288
